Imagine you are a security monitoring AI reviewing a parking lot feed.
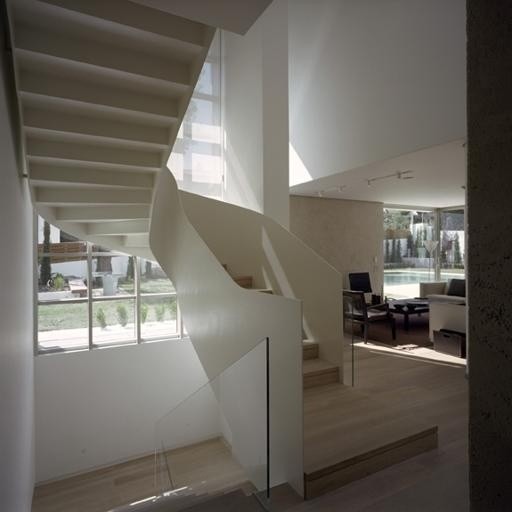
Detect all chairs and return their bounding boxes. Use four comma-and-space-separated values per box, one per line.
343, 290, 389, 344
428, 303, 467, 358
427, 278, 466, 301
414, 281, 446, 299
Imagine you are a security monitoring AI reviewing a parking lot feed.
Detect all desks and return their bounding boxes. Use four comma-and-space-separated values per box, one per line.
68, 279, 87, 297
